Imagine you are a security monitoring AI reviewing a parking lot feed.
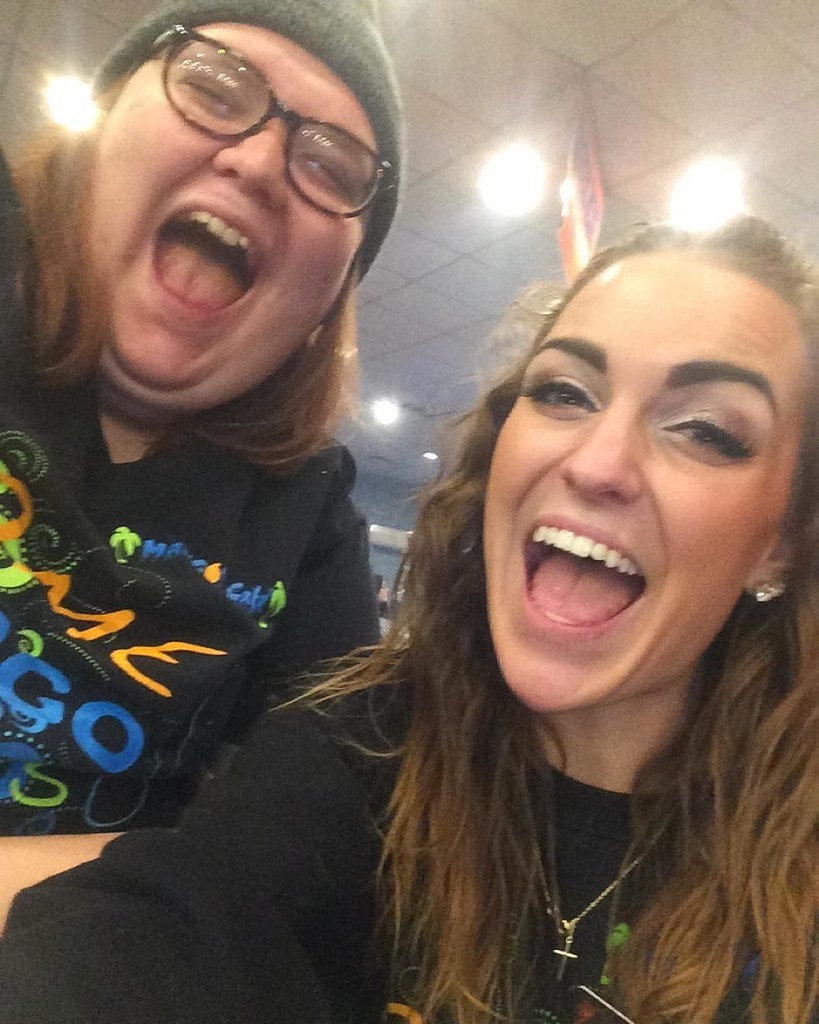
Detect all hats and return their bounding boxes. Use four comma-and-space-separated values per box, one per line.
90, 0, 405, 291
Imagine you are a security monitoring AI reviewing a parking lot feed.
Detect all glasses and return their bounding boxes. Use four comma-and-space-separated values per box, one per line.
123, 24, 392, 218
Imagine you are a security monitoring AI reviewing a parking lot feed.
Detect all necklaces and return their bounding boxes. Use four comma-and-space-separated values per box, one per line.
542, 828, 658, 982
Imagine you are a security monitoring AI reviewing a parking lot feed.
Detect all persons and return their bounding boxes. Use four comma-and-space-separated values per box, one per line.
0, 0, 403, 930
1, 208, 819, 1024
